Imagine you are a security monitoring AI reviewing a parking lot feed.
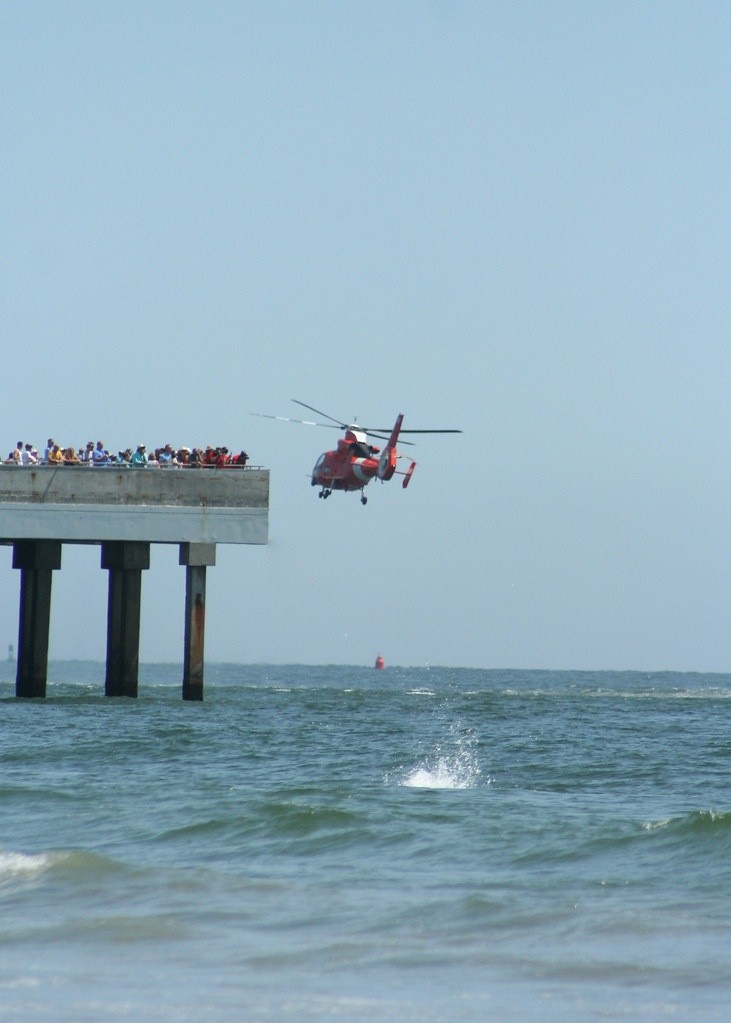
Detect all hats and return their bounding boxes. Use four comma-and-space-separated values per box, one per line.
181, 446, 187, 451
137, 444, 145, 451
206, 445, 214, 450
25, 444, 33, 448
32, 449, 37, 452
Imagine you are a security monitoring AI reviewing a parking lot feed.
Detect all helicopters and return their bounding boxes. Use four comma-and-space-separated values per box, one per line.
250, 399, 462, 505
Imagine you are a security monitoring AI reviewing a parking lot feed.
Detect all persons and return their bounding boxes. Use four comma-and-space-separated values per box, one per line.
82, 442, 103, 465
233, 450, 249, 469
93, 440, 111, 467
31, 449, 38, 459
22, 444, 36, 465
62, 448, 81, 465
13, 441, 23, 465
4, 452, 14, 464
104, 444, 148, 468
77, 446, 86, 461
48, 445, 63, 465
147, 444, 233, 468
45, 439, 54, 461
